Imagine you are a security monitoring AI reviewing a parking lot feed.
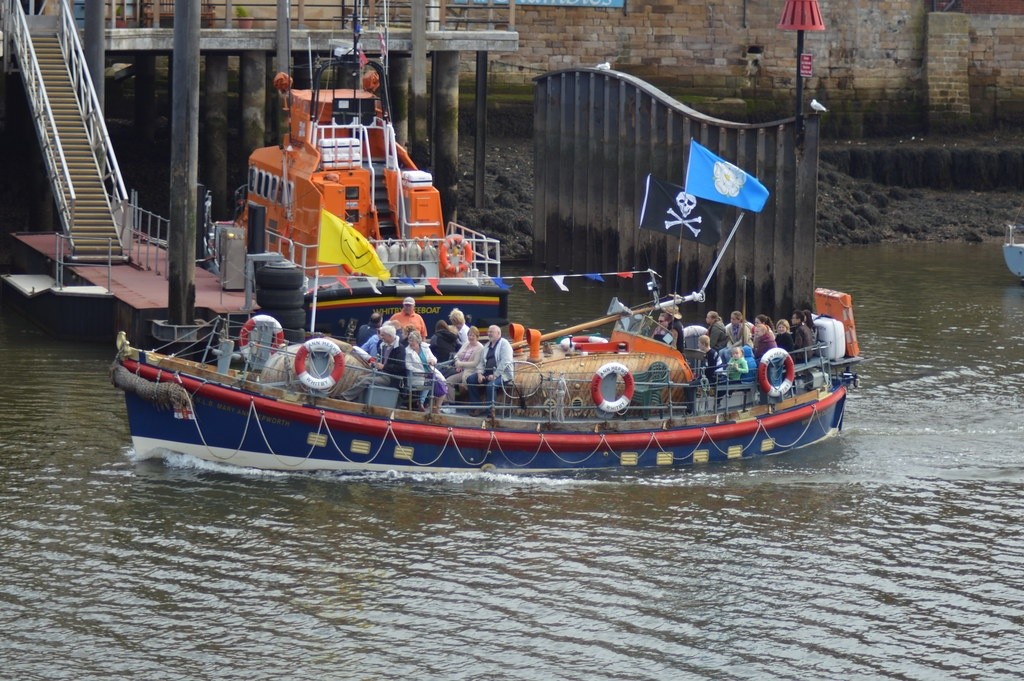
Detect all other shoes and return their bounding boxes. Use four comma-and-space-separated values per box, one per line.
416, 402, 425, 411
334, 395, 346, 400
469, 409, 485, 416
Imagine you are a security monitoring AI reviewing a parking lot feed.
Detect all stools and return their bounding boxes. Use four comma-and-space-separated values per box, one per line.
404, 385, 428, 409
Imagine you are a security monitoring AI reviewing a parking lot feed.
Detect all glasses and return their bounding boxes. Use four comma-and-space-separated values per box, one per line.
658, 320, 666, 323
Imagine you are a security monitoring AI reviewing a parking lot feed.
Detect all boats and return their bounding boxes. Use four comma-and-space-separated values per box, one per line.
204, 0, 510, 340
1002, 224, 1024, 283
107, 211, 861, 475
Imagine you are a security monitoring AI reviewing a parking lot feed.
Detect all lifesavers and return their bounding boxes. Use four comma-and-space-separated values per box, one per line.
239, 314, 284, 359
758, 348, 795, 397
294, 338, 344, 390
560, 336, 610, 354
591, 362, 634, 412
438, 233, 473, 274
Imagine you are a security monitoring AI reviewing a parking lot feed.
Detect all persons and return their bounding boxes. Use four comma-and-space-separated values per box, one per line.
651, 301, 819, 415
331, 296, 514, 415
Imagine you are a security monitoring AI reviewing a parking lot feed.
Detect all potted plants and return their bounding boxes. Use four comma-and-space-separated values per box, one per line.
236, 6, 252, 29
116, 5, 129, 29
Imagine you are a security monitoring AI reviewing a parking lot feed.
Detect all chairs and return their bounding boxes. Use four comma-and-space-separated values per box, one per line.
624, 362, 668, 420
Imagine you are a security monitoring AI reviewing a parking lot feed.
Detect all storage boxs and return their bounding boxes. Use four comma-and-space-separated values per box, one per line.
319, 138, 361, 167
403, 171, 434, 188
367, 386, 399, 407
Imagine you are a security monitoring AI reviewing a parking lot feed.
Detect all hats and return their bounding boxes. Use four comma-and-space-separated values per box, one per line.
403, 297, 415, 305
661, 304, 682, 319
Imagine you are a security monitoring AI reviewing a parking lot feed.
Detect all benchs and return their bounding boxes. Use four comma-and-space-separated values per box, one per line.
782, 341, 831, 397
143, 0, 218, 28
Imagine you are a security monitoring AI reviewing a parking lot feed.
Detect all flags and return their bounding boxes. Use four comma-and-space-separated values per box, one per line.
398, 276, 416, 286
378, 32, 386, 55
684, 140, 770, 213
637, 173, 722, 246
551, 275, 569, 291
521, 277, 536, 294
366, 277, 382, 294
492, 278, 514, 289
618, 272, 634, 278
359, 48, 368, 67
584, 273, 605, 281
427, 279, 443, 295
336, 276, 352, 294
317, 209, 390, 282
